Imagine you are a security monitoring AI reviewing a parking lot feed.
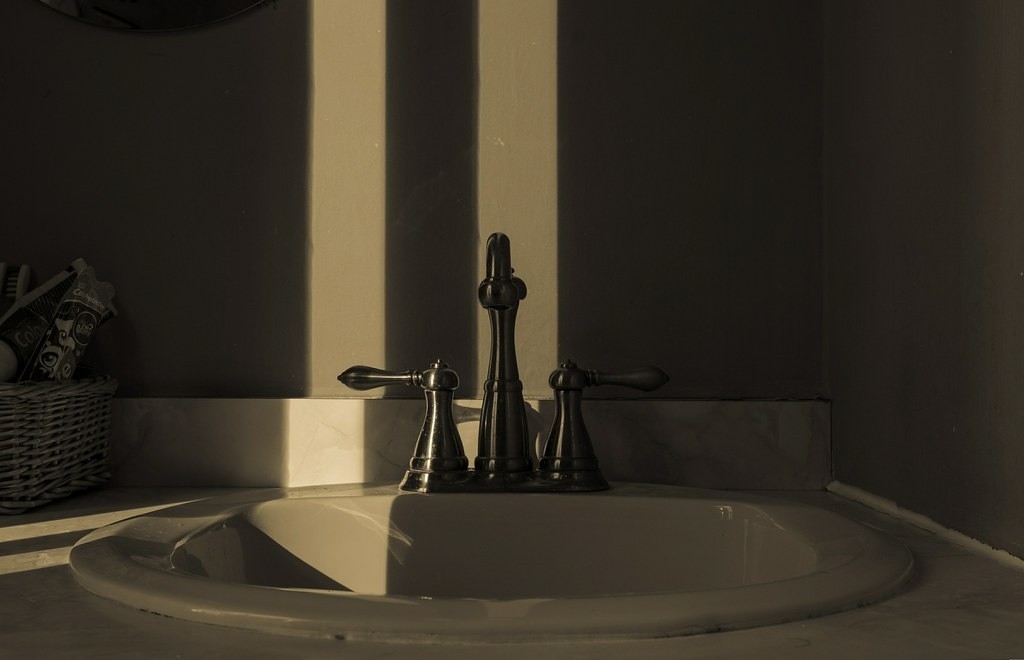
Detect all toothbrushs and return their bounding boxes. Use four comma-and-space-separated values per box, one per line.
5, 263, 33, 305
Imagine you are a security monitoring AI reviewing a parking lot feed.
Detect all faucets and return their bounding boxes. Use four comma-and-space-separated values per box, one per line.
470, 228, 535, 491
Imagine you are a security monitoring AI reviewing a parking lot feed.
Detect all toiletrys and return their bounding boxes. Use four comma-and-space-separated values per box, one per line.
15, 272, 114, 392
0, 259, 119, 385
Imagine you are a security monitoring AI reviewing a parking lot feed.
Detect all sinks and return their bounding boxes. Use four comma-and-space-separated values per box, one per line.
64, 474, 923, 649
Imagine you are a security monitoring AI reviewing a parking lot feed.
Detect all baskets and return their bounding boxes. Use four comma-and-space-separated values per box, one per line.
1, 373, 117, 509
